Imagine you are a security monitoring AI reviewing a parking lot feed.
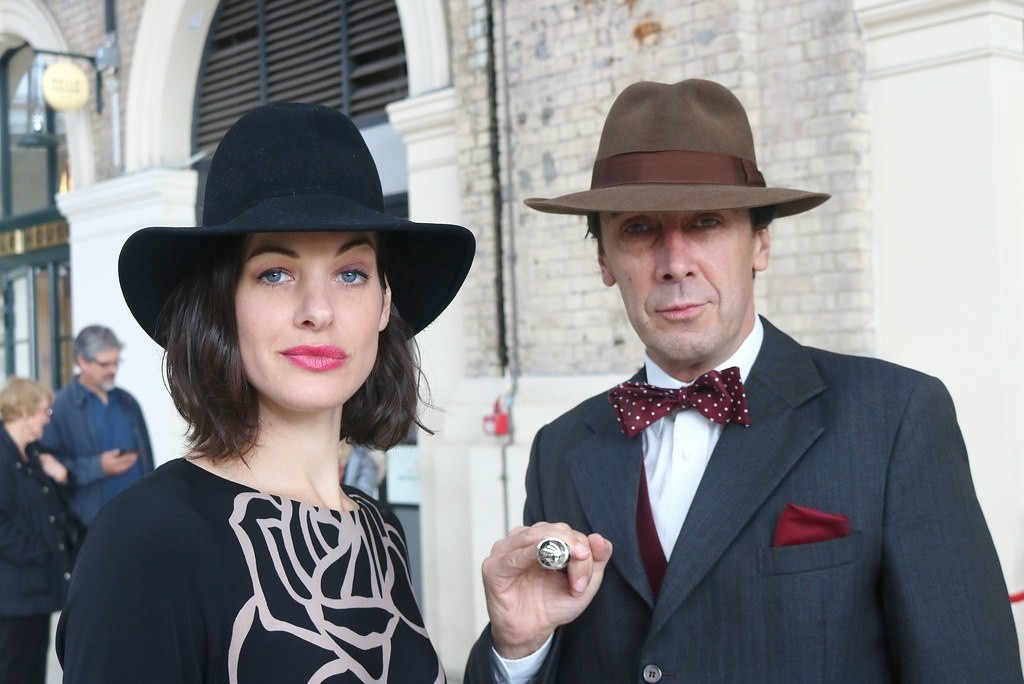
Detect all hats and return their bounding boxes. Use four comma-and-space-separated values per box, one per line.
117, 101, 472, 351
523, 79, 832, 218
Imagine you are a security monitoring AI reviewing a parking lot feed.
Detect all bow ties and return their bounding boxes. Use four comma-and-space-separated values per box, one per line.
608, 366, 752, 439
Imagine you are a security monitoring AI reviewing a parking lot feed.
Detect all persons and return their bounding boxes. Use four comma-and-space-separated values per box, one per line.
463, 78, 1024, 684
54, 97, 477, 684
0, 379, 88, 684
35, 325, 154, 529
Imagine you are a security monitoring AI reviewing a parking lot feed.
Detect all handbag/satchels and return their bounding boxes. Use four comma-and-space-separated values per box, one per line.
37, 445, 87, 549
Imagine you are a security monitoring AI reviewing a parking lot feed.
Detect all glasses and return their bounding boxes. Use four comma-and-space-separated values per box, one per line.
91, 357, 119, 370
39, 408, 53, 417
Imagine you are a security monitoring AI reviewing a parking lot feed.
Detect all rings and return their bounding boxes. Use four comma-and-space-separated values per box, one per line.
536, 537, 569, 570
